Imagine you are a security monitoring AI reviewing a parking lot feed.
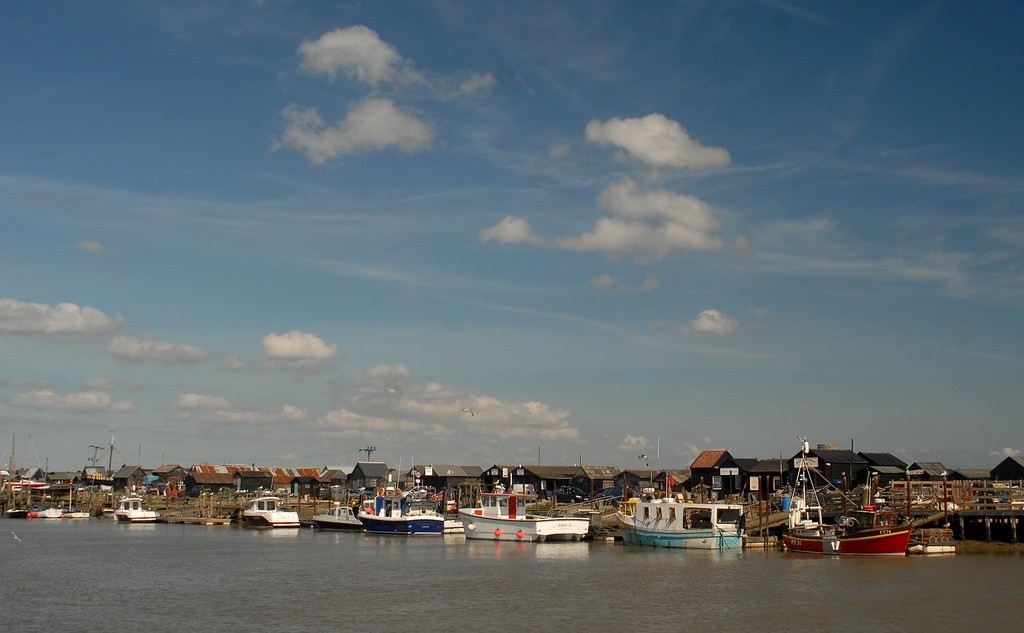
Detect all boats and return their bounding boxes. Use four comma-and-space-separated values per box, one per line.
356, 455, 445, 535
5, 430, 91, 518
102, 507, 114, 518
457, 493, 591, 543
244, 496, 301, 528
112, 497, 157, 522
780, 435, 912, 555
614, 501, 746, 550
312, 505, 364, 533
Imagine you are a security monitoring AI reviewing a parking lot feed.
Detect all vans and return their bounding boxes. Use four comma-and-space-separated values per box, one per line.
401, 485, 437, 499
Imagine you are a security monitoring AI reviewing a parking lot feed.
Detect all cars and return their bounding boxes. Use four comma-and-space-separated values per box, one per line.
595, 486, 636, 505
546, 486, 588, 503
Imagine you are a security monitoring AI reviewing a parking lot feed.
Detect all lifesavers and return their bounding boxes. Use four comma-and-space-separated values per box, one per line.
377, 488, 385, 496
395, 488, 402, 497
849, 521, 854, 527
864, 505, 876, 511
